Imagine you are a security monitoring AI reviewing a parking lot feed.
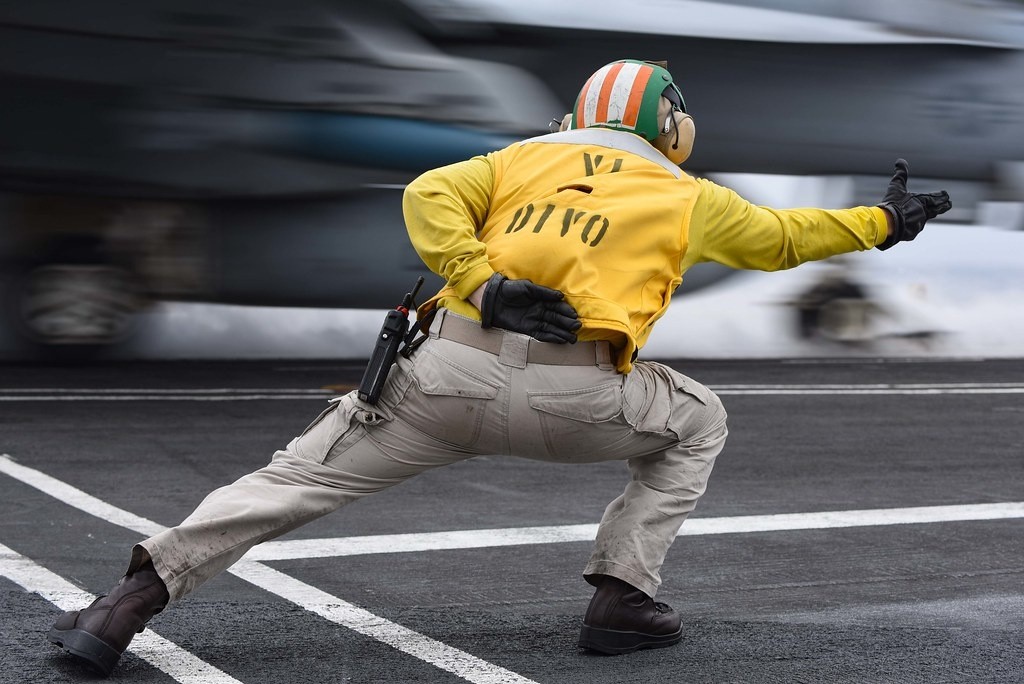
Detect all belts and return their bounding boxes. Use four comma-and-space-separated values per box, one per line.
428, 309, 617, 366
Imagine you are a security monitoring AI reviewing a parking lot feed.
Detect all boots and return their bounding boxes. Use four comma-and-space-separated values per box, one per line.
48, 566, 169, 674
579, 576, 682, 654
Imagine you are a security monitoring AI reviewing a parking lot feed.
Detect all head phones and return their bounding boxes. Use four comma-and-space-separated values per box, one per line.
558, 111, 695, 165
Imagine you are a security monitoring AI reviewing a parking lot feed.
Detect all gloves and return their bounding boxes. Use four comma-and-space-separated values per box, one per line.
875, 158, 953, 250
481, 271, 581, 345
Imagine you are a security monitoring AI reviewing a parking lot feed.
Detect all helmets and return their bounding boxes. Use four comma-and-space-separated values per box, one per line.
571, 59, 687, 143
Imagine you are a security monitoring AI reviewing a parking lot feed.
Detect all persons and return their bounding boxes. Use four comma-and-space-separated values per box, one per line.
42, 51, 953, 670
